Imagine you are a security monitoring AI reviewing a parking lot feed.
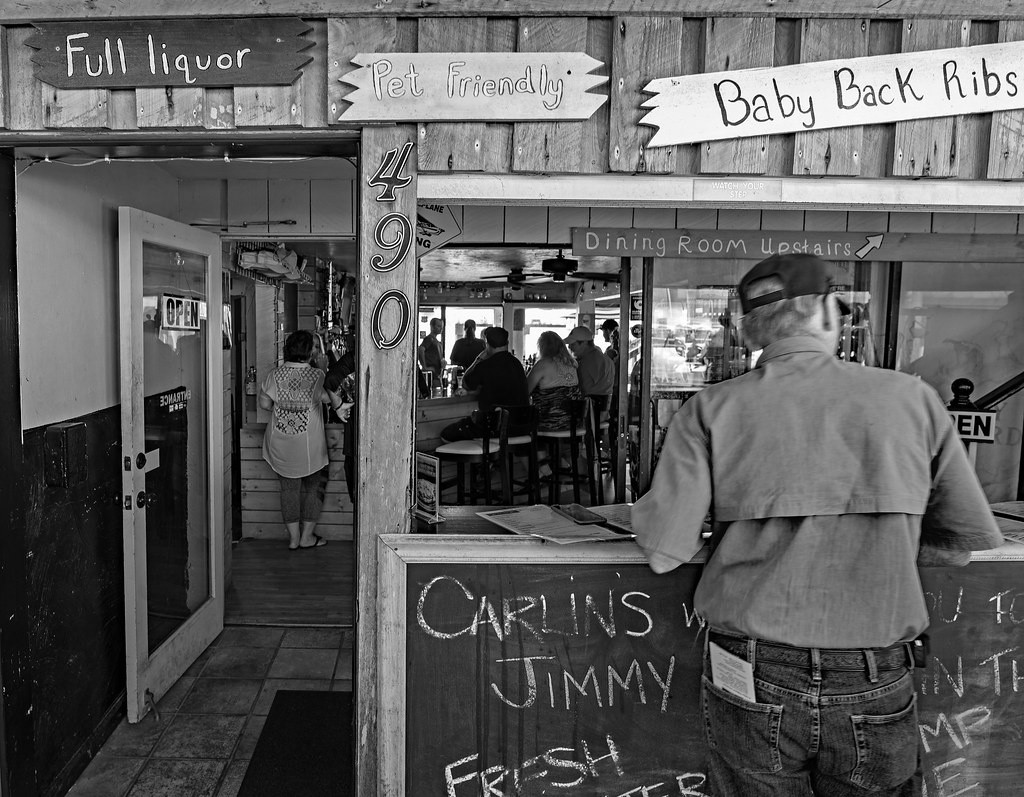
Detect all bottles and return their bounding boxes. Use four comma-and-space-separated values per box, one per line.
245, 366, 256, 396
522, 353, 537, 375
443, 371, 448, 389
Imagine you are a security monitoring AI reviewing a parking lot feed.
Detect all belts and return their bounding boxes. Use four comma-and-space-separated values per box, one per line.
714, 637, 908, 671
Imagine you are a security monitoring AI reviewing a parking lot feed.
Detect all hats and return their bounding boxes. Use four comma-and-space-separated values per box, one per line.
563, 326, 593, 344
739, 253, 851, 317
599, 319, 618, 330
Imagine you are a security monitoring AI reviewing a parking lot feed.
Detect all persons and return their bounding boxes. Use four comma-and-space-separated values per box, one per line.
257, 318, 620, 552
630, 253, 1006, 797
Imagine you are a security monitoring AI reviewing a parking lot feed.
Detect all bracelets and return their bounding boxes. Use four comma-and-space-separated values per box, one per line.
333, 401, 343, 411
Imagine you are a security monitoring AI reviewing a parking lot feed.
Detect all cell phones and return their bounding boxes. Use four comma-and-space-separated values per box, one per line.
551, 504, 607, 527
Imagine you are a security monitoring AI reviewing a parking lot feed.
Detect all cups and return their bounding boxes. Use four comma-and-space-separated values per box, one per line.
423, 372, 433, 391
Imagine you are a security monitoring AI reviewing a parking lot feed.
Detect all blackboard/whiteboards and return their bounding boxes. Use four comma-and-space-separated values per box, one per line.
375, 532, 1024, 797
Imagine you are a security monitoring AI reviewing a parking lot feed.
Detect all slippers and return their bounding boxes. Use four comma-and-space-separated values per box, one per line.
289, 545, 300, 550
300, 532, 327, 548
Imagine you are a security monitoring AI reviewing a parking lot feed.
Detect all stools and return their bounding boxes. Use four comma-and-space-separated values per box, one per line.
436, 394, 616, 506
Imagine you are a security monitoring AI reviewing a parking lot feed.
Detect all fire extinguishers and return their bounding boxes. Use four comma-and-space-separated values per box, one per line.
244, 366, 257, 412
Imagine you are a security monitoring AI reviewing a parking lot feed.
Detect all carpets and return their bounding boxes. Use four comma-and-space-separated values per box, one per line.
235, 690, 359, 797
